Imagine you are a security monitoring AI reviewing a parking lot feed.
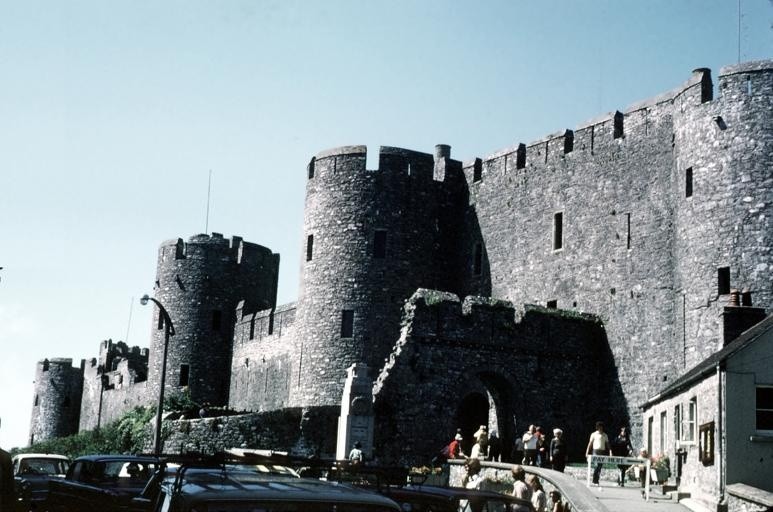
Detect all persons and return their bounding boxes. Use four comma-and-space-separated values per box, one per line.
349, 440, 363, 464
586, 421, 612, 486
610, 427, 634, 488
449, 421, 566, 512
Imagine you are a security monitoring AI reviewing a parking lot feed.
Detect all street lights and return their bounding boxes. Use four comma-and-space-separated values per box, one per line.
141, 295, 175, 455
